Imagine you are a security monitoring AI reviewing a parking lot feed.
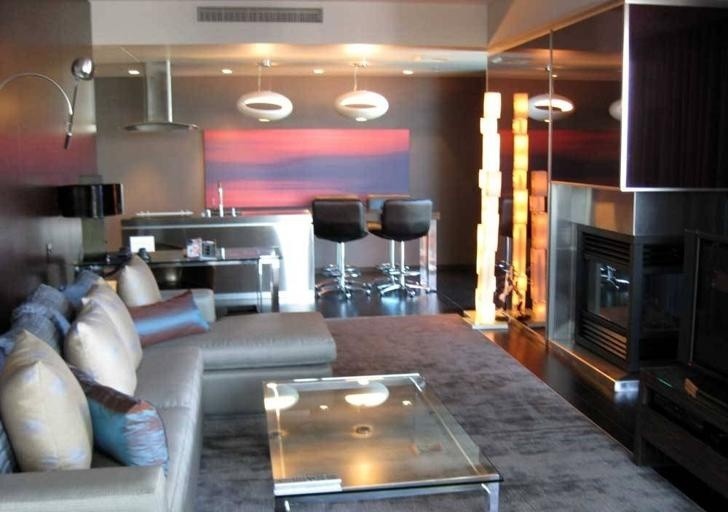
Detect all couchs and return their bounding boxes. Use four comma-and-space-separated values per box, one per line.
0, 274, 338, 510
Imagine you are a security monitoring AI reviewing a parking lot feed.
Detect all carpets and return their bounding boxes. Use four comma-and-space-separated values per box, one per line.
196, 310, 702, 512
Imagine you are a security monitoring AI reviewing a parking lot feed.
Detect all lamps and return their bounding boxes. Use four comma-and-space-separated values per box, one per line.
1, 55, 95, 150
236, 59, 292, 124
334, 61, 388, 123
56, 172, 127, 268
525, 62, 572, 123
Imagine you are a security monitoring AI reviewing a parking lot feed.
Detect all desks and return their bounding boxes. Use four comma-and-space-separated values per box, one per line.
634, 362, 726, 510
78, 244, 282, 313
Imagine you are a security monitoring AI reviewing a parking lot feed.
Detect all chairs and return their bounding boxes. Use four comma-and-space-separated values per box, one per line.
313, 195, 371, 302
368, 193, 431, 298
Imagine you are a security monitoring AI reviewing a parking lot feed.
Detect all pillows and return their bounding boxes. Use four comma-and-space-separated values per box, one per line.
81, 277, 145, 371
66, 361, 170, 467
1, 328, 94, 470
127, 289, 211, 349
118, 254, 163, 308
64, 299, 137, 399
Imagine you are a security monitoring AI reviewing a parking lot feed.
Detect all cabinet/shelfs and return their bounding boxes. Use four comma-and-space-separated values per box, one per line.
552, 0, 728, 195
